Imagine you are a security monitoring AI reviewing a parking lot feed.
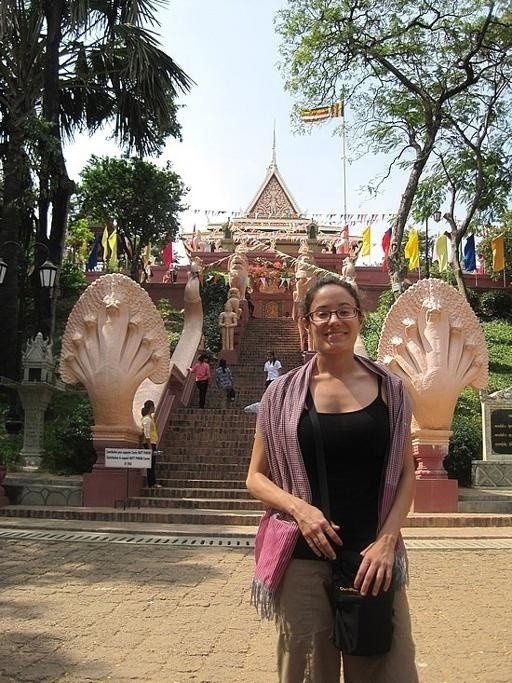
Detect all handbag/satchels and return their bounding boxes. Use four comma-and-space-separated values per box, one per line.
323, 550, 394, 656
230, 389, 235, 397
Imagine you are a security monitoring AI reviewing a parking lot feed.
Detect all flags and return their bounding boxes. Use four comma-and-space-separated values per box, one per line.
88, 223, 174, 279
337, 221, 507, 274
299, 102, 343, 122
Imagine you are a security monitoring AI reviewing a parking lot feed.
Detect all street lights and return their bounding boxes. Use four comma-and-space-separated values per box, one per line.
424, 205, 442, 279
0, 239, 57, 436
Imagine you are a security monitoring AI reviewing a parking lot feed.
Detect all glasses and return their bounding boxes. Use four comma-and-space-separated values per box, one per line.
305, 308, 362, 323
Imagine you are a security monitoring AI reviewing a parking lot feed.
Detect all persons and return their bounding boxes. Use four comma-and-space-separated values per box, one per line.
244, 287, 258, 318
188, 354, 211, 408
247, 278, 421, 682
140, 400, 164, 488
215, 358, 237, 406
262, 351, 283, 384
169, 261, 181, 280
144, 260, 152, 278
